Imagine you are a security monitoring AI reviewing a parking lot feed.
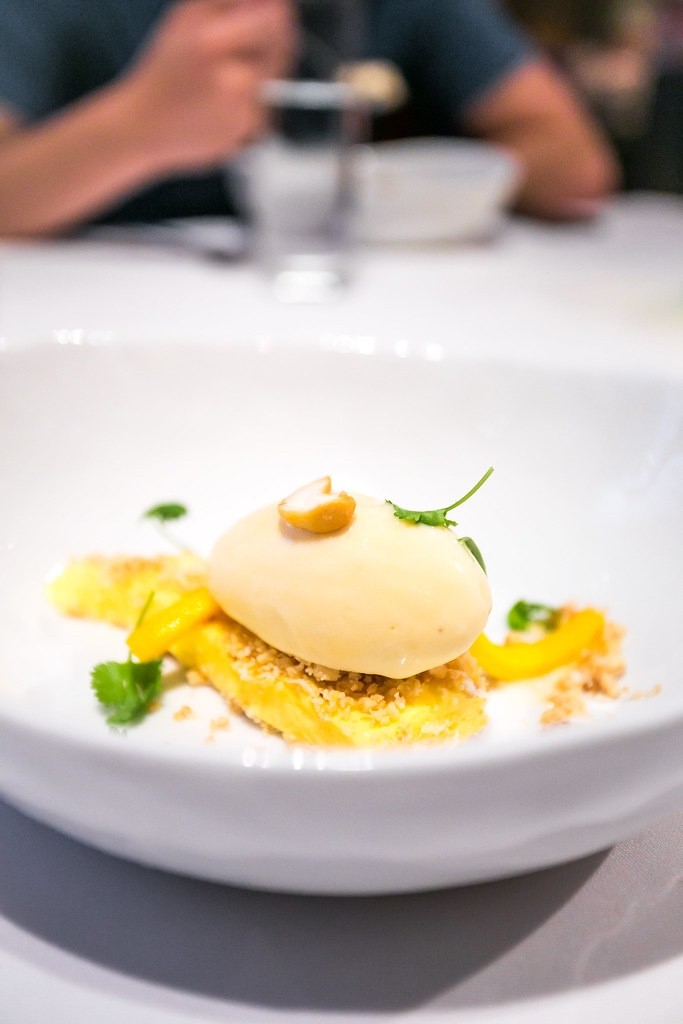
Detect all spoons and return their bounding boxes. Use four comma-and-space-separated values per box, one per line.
261, 63, 406, 111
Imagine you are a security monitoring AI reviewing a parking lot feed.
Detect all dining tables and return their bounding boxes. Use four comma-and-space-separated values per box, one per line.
0, 196, 683, 1024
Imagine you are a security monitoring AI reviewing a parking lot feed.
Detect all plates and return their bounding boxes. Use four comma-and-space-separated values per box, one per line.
0, 335, 683, 897
237, 141, 524, 250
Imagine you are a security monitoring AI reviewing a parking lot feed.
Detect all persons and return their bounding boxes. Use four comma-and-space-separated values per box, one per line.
0, 0, 621, 241
